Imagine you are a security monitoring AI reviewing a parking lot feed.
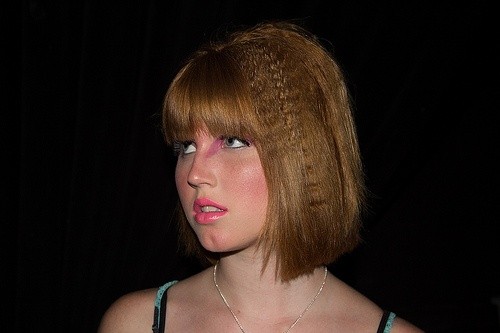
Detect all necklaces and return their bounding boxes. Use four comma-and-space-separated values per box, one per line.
212, 258, 329, 332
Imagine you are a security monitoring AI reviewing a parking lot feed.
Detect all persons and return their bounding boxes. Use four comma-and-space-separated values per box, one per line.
95, 21, 427, 333
